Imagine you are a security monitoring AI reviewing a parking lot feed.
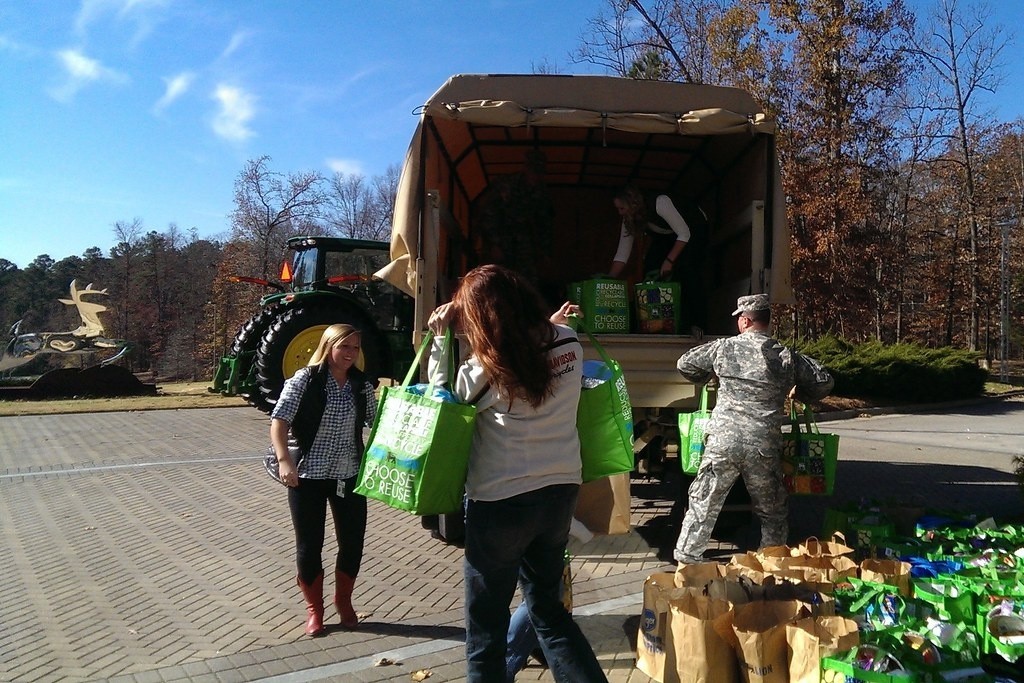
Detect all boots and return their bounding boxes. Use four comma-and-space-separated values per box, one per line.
297, 569, 325, 638
335, 569, 358, 629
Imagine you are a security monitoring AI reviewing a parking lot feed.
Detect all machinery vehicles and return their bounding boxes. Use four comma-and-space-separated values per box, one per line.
207, 237, 415, 422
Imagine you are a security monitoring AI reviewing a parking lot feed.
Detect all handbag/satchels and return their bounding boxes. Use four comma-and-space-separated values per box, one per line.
678, 384, 713, 476
352, 326, 477, 516
573, 471, 631, 537
635, 270, 682, 335
421, 320, 467, 549
775, 399, 839, 497
634, 519, 1023, 682
568, 273, 630, 334
567, 313, 635, 483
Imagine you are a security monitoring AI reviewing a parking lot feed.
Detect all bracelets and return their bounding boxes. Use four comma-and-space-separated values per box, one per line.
665, 256, 675, 266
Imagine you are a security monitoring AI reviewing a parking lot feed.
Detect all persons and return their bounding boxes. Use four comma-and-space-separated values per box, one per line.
673, 293, 835, 570
609, 190, 714, 335
427, 265, 611, 683
271, 324, 379, 636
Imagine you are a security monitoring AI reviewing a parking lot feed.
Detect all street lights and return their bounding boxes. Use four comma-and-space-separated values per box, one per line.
208, 301, 216, 381
995, 219, 1020, 381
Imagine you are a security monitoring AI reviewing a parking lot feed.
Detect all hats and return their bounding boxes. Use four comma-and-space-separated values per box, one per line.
731, 293, 771, 317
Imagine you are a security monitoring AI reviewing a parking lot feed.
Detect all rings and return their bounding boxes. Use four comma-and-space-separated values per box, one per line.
432, 310, 438, 315
283, 479, 287, 483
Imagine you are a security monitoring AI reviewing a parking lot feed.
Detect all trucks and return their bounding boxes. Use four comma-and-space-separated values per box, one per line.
375, 73, 795, 477
